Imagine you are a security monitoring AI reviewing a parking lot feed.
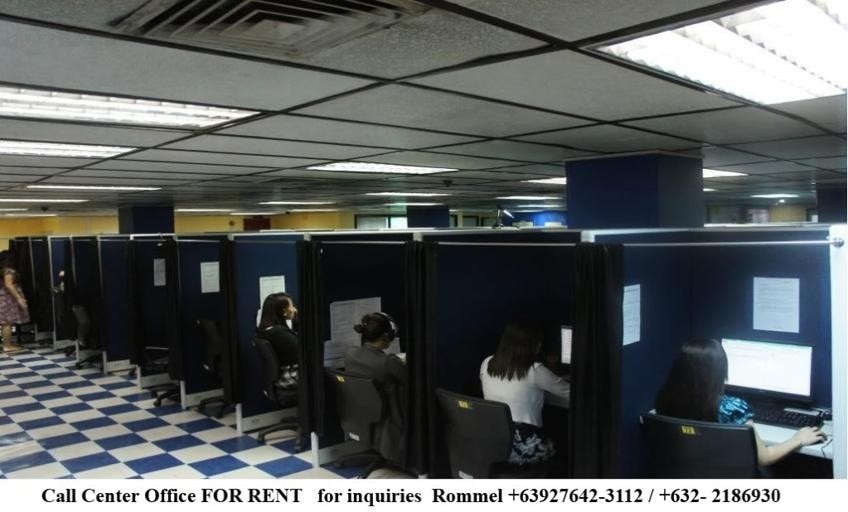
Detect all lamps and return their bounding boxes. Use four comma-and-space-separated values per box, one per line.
494, 204, 515, 227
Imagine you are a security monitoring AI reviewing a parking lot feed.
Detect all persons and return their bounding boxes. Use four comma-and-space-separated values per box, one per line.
0, 250, 31, 353
478, 315, 570, 472
256, 292, 300, 409
654, 336, 828, 473
343, 311, 407, 465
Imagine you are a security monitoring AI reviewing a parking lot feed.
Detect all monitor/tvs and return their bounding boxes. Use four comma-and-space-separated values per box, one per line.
720, 337, 818, 410
743, 207, 771, 224
560, 326, 573, 365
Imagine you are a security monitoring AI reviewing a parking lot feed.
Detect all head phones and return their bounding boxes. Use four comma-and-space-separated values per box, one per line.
375, 311, 398, 342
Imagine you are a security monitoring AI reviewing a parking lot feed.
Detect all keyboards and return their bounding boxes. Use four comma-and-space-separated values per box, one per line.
752, 407, 824, 429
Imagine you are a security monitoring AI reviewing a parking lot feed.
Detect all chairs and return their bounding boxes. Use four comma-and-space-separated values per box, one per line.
12, 291, 762, 479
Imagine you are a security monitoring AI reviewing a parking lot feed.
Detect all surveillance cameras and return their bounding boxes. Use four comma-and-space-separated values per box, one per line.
443, 180, 453, 187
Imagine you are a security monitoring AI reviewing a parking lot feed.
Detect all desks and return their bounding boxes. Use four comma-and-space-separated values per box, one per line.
751, 417, 833, 460
